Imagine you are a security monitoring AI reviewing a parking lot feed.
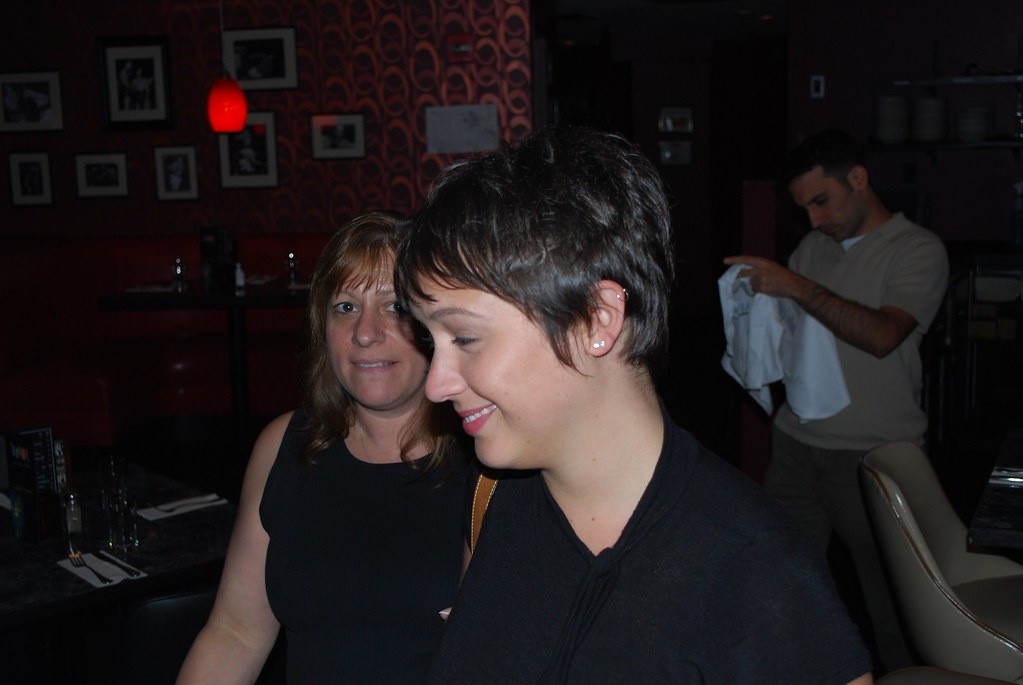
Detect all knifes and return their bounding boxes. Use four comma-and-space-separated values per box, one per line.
88, 547, 140, 578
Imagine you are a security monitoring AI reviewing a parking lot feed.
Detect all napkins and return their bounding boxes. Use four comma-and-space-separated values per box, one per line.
135, 493, 228, 522
56, 549, 148, 590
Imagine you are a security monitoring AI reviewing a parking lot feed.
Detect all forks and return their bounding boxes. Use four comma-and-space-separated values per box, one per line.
148, 498, 223, 513
67, 546, 113, 584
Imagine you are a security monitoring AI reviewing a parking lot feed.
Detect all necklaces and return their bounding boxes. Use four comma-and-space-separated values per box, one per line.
357, 413, 421, 461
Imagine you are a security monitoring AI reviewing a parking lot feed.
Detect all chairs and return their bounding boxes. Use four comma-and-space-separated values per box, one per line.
857, 440, 1023, 685
126, 413, 273, 499
118, 590, 216, 685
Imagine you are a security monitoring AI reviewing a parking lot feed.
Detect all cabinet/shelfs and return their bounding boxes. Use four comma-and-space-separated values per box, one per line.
857, 73, 1023, 152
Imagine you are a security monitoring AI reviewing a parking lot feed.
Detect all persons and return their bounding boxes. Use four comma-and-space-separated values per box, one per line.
2, 85, 51, 123
88, 166, 116, 186
175, 210, 475, 685
26, 165, 43, 194
233, 44, 247, 79
330, 125, 354, 148
120, 60, 149, 110
236, 129, 261, 175
392, 124, 874, 685
723, 134, 949, 675
168, 155, 184, 190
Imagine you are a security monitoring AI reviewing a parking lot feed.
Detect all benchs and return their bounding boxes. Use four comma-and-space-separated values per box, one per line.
0, 233, 330, 449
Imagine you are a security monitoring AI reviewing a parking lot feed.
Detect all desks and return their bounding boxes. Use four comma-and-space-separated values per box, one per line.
96, 276, 310, 463
966, 426, 1023, 564
0, 456, 238, 685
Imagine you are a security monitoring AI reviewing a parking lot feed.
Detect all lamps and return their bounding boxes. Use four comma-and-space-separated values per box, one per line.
207, 0, 249, 134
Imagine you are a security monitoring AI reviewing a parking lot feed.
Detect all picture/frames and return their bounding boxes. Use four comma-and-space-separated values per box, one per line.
94, 33, 177, 134
306, 112, 367, 160
219, 25, 302, 91
73, 150, 129, 200
6, 149, 56, 208
217, 109, 279, 190
0, 68, 65, 134
152, 143, 201, 204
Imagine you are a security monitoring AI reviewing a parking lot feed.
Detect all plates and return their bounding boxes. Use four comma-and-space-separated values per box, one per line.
877, 97, 909, 144
957, 127, 985, 142
913, 101, 945, 142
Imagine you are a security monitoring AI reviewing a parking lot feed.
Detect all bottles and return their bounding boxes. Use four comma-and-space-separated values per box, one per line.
101, 453, 138, 547
236, 263, 245, 297
45, 443, 82, 557
289, 250, 298, 287
174, 258, 187, 300
